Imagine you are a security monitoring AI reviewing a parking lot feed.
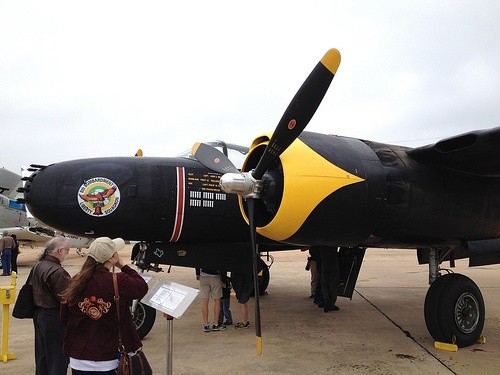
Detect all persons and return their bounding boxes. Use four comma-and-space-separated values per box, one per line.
0, 229, 20, 276
64, 236, 150, 375
193, 241, 254, 332
33, 236, 76, 375
300, 243, 345, 312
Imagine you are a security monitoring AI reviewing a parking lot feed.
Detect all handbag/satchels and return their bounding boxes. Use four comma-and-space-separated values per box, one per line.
13, 264, 35, 318
118, 351, 152, 375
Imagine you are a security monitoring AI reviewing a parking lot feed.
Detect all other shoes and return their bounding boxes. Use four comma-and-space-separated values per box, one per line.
203, 325, 210, 332
224, 320, 232, 325
219, 325, 226, 329
0, 274, 11, 276
235, 321, 249, 328
310, 294, 339, 313
212, 325, 218, 330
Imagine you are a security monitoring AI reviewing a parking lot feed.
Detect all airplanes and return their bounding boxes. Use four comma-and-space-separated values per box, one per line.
13, 47, 500, 356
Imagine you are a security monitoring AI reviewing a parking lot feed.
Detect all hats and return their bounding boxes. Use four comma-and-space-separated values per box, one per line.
88, 237, 125, 262
